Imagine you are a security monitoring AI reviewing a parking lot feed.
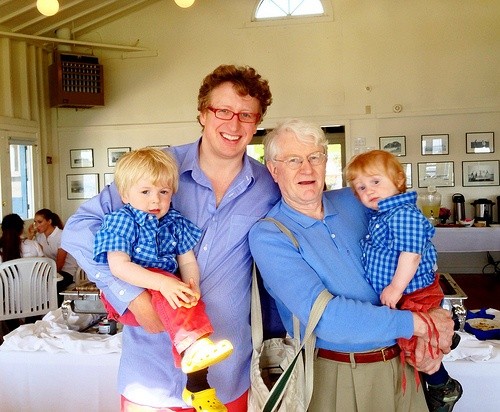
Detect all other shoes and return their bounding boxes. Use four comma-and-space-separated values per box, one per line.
427, 378, 463, 412
450, 333, 461, 350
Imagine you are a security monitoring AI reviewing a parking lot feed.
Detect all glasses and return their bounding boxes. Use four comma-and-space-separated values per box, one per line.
207, 106, 261, 123
275, 151, 328, 170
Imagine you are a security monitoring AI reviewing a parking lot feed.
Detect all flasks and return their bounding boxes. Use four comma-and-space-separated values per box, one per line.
452, 192, 466, 223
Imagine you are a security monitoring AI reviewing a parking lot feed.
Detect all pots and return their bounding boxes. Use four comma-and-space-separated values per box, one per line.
470, 198, 496, 224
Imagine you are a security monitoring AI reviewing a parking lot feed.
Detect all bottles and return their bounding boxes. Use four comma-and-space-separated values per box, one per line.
422, 190, 441, 219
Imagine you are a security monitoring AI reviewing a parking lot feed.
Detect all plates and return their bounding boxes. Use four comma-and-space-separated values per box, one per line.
465, 318, 500, 332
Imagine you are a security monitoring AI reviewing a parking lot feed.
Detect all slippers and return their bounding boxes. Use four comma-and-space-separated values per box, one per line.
182, 387, 228, 412
181, 338, 233, 373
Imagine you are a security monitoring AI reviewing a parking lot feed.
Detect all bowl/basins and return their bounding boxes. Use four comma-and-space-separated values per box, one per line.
460, 219, 475, 227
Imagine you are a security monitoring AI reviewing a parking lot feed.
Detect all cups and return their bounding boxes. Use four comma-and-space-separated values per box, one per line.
31, 221, 41, 233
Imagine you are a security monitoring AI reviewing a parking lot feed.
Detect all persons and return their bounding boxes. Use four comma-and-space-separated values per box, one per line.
344, 150, 464, 412
0, 208, 77, 330
93, 147, 233, 412
62, 65, 283, 412
248, 119, 455, 412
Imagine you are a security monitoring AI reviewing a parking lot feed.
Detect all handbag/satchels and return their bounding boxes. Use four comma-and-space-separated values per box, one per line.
247, 217, 335, 412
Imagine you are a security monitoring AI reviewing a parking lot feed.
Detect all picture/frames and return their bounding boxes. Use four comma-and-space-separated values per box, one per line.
103, 173, 115, 187
107, 146, 131, 167
465, 131, 495, 154
69, 148, 95, 168
421, 133, 449, 155
379, 136, 406, 157
402, 163, 412, 189
417, 161, 455, 188
66, 173, 99, 200
462, 160, 500, 187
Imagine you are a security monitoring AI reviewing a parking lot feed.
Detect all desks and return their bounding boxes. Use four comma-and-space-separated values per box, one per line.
0, 308, 500, 412
431, 224, 500, 280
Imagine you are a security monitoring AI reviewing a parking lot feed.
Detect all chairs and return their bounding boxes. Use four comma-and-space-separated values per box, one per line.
0, 257, 58, 321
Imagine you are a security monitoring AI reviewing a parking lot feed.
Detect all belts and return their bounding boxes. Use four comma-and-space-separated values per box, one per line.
317, 345, 400, 364
